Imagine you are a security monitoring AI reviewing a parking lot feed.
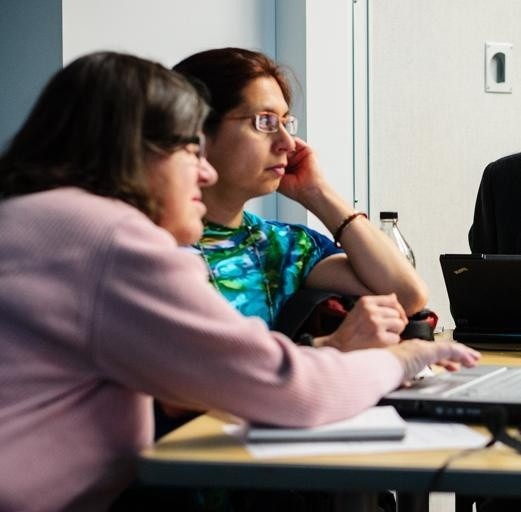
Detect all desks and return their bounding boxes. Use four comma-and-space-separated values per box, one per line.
122, 327, 520, 510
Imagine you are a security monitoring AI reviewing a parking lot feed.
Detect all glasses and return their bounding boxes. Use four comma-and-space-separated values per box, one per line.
153, 131, 206, 155
228, 109, 298, 135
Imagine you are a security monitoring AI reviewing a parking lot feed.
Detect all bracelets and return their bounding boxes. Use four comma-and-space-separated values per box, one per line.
333, 208, 368, 247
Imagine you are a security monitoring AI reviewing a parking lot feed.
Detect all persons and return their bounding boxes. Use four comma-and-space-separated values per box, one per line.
0, 51, 482, 512
152, 46, 430, 430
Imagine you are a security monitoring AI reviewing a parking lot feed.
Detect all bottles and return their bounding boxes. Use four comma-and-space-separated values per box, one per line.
378, 211, 415, 270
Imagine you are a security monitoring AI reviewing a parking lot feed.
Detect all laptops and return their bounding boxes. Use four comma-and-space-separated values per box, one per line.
440, 254, 521, 349
377, 365, 521, 428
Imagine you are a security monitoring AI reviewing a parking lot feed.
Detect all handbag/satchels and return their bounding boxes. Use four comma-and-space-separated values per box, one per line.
275, 290, 437, 341
